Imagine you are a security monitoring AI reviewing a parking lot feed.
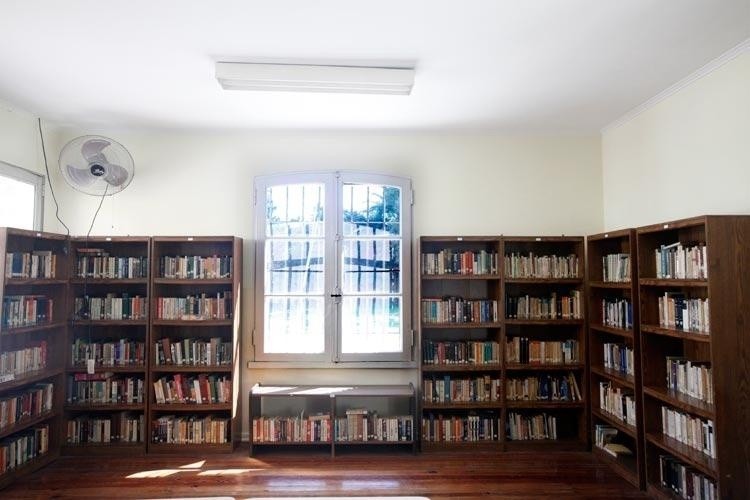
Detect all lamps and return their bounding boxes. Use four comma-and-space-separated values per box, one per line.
215, 62, 416, 96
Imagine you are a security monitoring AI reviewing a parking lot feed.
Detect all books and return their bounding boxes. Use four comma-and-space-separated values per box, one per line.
655, 242, 717, 499
595, 254, 636, 456
152, 256, 233, 443
504, 252, 582, 441
253, 408, 414, 443
68, 248, 147, 442
420, 250, 502, 441
1, 248, 56, 471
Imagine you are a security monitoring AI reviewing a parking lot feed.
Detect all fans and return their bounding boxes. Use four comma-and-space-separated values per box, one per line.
58, 134, 134, 198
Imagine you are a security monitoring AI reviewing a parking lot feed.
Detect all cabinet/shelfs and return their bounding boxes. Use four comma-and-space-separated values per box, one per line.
0, 226, 76, 489
635, 215, 750, 500
503, 235, 590, 449
417, 236, 505, 451
249, 381, 419, 457
586, 227, 643, 490
149, 236, 242, 454
71, 235, 150, 455
